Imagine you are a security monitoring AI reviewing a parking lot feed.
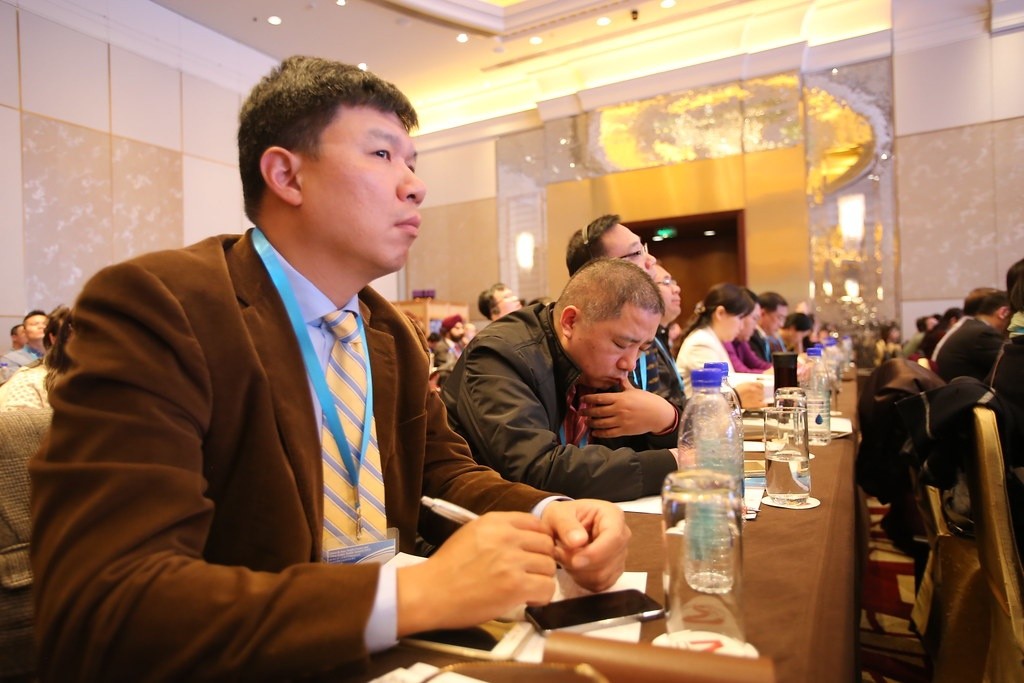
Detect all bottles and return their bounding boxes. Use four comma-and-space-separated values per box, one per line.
800, 335, 850, 446
703, 361, 743, 460
677, 365, 743, 592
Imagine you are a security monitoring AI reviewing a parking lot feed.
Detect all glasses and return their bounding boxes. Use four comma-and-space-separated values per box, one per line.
656, 278, 677, 286
618, 242, 649, 259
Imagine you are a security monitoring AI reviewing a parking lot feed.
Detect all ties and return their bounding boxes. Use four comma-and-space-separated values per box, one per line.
320, 309, 393, 559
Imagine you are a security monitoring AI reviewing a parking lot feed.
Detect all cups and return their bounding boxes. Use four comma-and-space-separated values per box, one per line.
663, 473, 744, 644
775, 387, 809, 459
763, 408, 811, 506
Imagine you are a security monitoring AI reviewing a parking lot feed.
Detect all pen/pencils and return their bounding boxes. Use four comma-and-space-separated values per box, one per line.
421, 495, 477, 525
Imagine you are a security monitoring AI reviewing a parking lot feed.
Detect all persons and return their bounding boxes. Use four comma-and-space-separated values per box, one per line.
0, 304, 73, 385
872, 321, 902, 367
438, 254, 702, 503
23, 53, 631, 683
899, 257, 1024, 566
427, 313, 479, 374
676, 282, 810, 399
478, 283, 524, 320
770, 299, 829, 355
565, 215, 768, 453
747, 290, 804, 367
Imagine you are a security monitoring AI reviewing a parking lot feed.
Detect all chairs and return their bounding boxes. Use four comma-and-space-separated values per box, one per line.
893, 376, 1024, 683
857, 358, 991, 683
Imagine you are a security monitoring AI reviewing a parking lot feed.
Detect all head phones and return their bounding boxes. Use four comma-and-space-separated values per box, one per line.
485, 288, 500, 315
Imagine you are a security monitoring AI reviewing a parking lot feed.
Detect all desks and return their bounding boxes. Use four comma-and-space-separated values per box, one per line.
314, 363, 872, 683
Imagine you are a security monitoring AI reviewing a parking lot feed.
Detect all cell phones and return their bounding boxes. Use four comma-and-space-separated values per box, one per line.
525, 589, 666, 637
401, 619, 534, 663
